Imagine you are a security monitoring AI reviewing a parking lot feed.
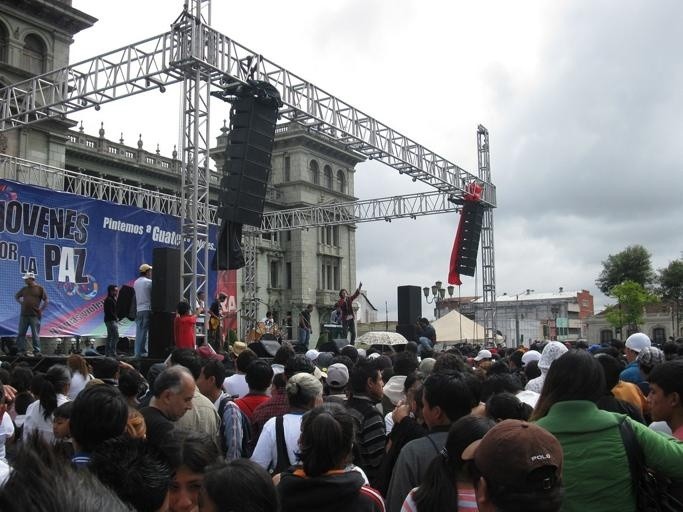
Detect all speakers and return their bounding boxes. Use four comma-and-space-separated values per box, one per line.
148, 310, 175, 360
319, 339, 350, 356
151, 248, 180, 312
247, 340, 282, 357
398, 286, 422, 326
455, 201, 485, 276
395, 325, 420, 344
216, 96, 279, 228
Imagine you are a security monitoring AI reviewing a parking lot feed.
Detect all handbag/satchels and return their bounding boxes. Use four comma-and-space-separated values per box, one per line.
617, 419, 683, 512
219, 397, 254, 458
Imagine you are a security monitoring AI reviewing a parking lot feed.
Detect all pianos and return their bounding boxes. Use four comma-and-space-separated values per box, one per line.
322, 323, 353, 340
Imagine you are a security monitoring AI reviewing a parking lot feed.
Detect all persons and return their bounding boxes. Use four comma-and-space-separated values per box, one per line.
337, 282, 363, 345
207, 293, 234, 346
0, 330, 682, 512
298, 304, 313, 348
259, 312, 273, 326
330, 304, 341, 339
415, 319, 435, 353
173, 301, 203, 351
14, 272, 48, 358
133, 263, 151, 357
196, 292, 205, 313
103, 284, 121, 358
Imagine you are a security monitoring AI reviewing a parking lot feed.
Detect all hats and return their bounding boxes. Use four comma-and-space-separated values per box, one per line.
197, 344, 225, 363
521, 350, 541, 367
636, 346, 666, 368
462, 419, 564, 490
139, 264, 153, 273
305, 349, 319, 360
326, 363, 349, 388
624, 333, 651, 353
474, 350, 492, 362
232, 341, 247, 357
22, 273, 35, 279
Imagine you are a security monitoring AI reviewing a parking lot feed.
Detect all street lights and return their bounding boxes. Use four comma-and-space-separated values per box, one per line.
514, 288, 535, 348
424, 281, 454, 320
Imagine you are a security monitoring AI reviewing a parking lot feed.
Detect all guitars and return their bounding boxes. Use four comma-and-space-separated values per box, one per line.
260, 319, 282, 336
210, 305, 240, 331
